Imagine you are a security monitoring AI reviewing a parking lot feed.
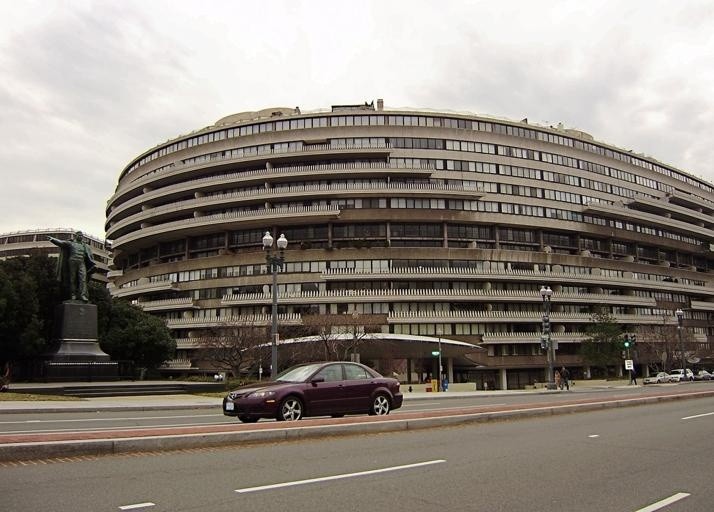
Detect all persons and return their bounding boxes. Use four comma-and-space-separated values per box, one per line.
629, 369, 637, 385
554, 370, 561, 390
45, 230, 97, 302
559, 367, 570, 391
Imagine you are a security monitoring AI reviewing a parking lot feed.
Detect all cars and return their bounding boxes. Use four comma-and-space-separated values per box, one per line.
694, 370, 714, 380
643, 372, 674, 384
223, 361, 403, 422
669, 368, 694, 382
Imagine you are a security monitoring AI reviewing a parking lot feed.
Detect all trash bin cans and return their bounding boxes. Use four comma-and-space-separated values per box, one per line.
136, 368, 144, 379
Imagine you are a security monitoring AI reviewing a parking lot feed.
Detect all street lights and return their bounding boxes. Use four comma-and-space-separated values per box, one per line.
540, 285, 557, 389
262, 231, 288, 377
675, 308, 688, 383
437, 328, 442, 392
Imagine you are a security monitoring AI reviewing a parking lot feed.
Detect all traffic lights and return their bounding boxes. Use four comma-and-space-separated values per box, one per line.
630, 333, 637, 347
624, 334, 629, 350
